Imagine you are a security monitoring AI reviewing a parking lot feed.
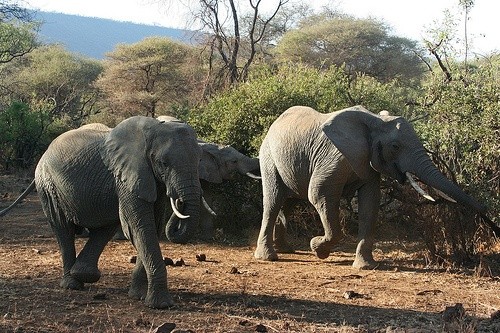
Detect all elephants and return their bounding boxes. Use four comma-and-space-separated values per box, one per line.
253, 105, 478, 270
1, 115, 263, 309
